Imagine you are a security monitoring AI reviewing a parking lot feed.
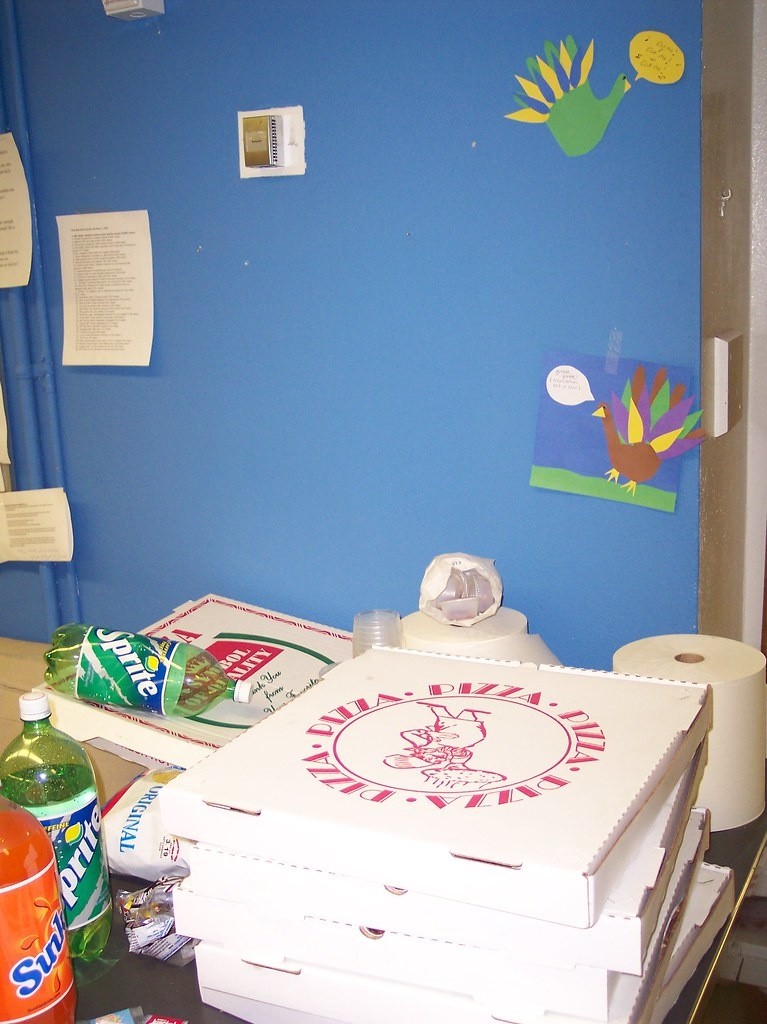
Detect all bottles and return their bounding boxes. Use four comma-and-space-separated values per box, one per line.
43, 624, 255, 717
0, 693, 113, 960
0, 779, 81, 1024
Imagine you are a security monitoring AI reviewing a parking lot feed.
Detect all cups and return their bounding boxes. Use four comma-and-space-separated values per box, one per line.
353, 609, 401, 660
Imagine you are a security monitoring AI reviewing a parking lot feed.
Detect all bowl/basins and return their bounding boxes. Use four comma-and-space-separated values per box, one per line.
429, 567, 493, 621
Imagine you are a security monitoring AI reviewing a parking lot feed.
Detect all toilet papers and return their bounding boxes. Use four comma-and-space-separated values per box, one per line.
401, 607, 563, 666
612, 634, 766, 832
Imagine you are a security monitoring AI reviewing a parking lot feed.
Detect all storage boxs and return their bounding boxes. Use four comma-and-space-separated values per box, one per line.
33, 591, 738, 1024
